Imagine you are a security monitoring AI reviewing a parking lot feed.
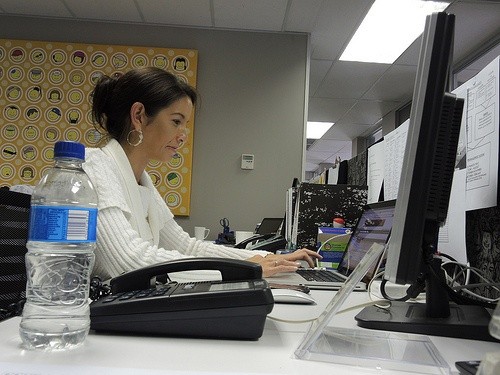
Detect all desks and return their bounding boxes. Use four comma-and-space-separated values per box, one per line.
0, 241, 500, 374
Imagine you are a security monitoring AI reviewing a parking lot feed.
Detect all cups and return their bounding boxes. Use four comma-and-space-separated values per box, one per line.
194, 227, 210, 240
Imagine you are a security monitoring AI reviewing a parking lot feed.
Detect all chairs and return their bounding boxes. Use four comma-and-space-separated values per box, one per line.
0, 188, 31, 319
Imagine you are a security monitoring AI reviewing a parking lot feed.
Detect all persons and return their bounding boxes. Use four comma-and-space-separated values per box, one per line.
65, 67, 323, 283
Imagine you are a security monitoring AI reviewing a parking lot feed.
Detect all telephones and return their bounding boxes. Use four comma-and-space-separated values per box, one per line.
234, 233, 287, 255
89, 257, 275, 342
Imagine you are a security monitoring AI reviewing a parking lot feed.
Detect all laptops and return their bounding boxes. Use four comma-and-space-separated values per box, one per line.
295, 199, 398, 290
256, 217, 284, 235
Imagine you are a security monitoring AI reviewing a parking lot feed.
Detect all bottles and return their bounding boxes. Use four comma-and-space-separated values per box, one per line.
19, 141, 99, 352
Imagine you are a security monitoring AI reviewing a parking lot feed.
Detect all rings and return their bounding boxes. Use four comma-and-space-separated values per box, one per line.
275, 262, 277, 266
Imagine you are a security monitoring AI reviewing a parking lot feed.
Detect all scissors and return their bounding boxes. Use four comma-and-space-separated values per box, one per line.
220, 217, 229, 228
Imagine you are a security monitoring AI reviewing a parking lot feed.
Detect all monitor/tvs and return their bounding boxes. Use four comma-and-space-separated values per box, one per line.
358, 10, 496, 343
